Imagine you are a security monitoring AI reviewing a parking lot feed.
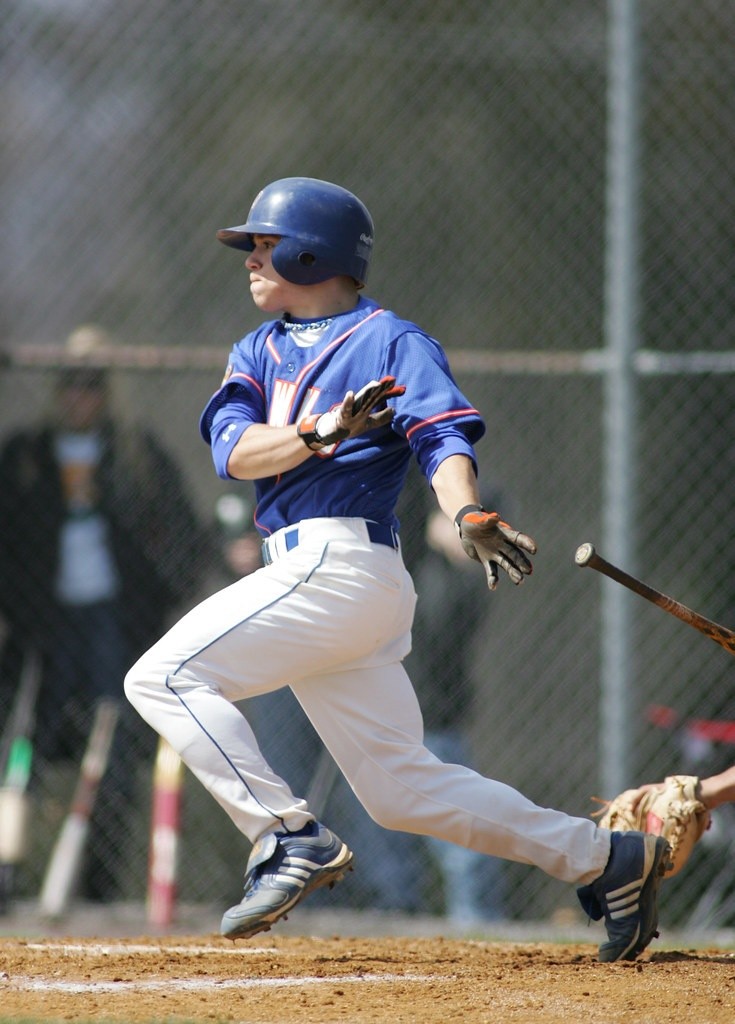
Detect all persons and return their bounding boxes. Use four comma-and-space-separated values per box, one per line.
122, 175, 676, 965
208, 455, 515, 920
0, 366, 200, 903
610, 765, 735, 878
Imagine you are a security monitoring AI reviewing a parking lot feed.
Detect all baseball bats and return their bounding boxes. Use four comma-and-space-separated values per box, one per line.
574, 541, 735, 659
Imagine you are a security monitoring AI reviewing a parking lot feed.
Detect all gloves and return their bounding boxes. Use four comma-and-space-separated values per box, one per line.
297, 376, 406, 451
453, 506, 536, 590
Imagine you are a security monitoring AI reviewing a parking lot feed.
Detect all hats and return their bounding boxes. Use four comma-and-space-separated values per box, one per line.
42, 325, 118, 375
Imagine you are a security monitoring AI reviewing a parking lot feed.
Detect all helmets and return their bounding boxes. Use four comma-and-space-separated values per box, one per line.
216, 177, 374, 289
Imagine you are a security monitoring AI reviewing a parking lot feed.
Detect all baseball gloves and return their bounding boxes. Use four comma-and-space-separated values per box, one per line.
597, 770, 714, 883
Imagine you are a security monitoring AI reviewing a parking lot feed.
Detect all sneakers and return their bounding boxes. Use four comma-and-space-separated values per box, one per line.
577, 831, 671, 962
220, 819, 355, 940
